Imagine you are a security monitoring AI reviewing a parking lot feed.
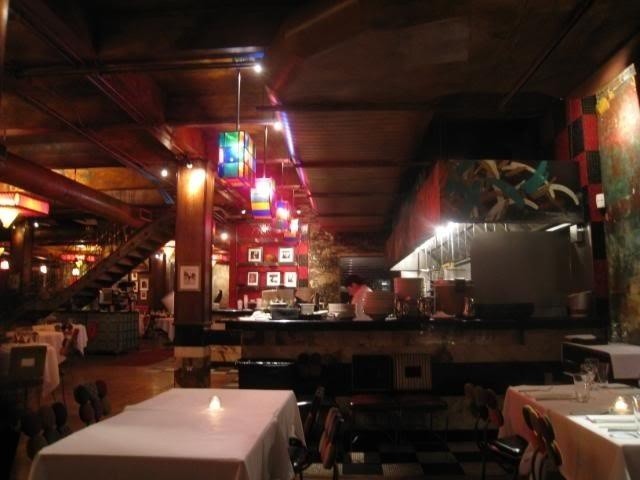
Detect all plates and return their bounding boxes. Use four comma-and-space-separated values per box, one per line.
328, 303, 357, 319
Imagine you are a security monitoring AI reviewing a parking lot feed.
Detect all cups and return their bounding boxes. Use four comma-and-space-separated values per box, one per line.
572, 373, 590, 404
631, 393, 640, 440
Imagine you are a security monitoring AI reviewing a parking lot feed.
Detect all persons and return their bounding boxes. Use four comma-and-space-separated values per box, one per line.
343, 274, 375, 319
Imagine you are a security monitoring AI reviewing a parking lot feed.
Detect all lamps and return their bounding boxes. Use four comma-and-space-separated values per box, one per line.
218, 63, 318, 241
0, 191, 50, 229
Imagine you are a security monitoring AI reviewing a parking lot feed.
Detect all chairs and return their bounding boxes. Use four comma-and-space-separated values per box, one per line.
23, 402, 73, 460
518, 405, 564, 479
74, 379, 110, 427
285, 405, 357, 479
466, 384, 528, 480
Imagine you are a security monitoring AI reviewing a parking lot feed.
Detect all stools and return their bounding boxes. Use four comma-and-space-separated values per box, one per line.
383, 392, 448, 449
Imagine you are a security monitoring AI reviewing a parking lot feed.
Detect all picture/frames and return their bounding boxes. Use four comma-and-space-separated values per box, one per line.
247, 247, 299, 289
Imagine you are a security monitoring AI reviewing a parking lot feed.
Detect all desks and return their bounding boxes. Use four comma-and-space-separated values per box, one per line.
139, 314, 174, 342
498, 383, 640, 480
28, 388, 307, 479
560, 341, 640, 381
1, 323, 89, 408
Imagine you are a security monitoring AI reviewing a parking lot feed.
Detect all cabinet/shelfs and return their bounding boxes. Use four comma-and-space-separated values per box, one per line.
236, 260, 307, 291
